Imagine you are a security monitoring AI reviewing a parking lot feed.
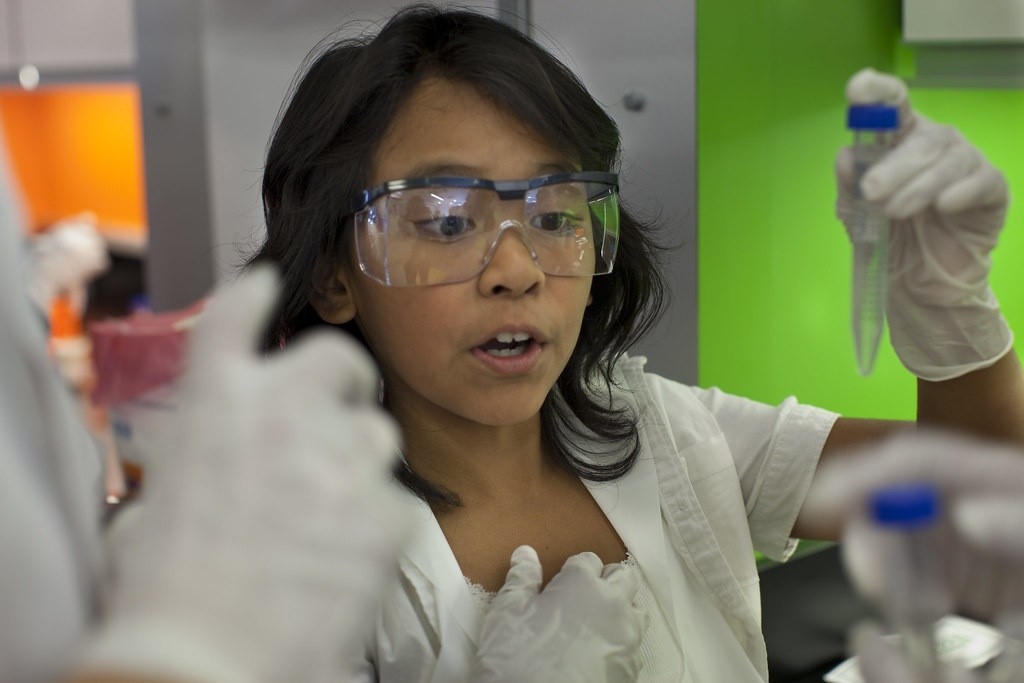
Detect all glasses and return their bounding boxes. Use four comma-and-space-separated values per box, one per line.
336, 170, 621, 288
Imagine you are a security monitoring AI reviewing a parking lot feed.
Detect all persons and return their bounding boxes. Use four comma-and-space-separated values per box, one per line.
1, 144, 416, 683
234, 5, 1024, 683
